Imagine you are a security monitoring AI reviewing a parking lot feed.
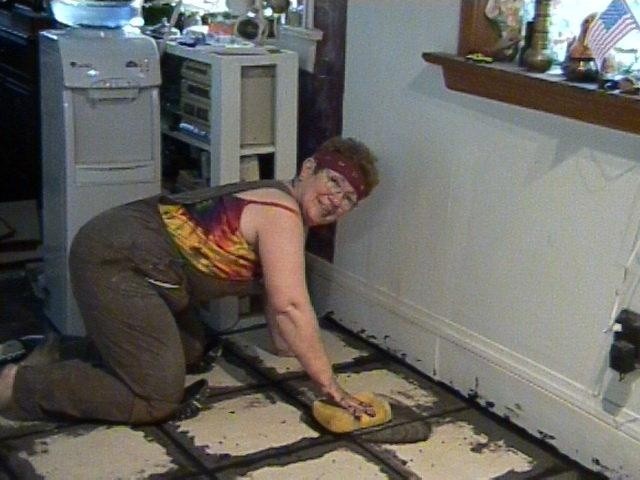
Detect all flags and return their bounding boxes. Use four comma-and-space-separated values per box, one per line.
583, 0, 640, 71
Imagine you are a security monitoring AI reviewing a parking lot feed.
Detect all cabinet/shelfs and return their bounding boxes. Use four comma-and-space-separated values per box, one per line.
0, 29, 39, 201
156, 40, 299, 334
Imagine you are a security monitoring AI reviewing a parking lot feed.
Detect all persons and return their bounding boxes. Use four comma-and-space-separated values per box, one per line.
0, 135, 381, 424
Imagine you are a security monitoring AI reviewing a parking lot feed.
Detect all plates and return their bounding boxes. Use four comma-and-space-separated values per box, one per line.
215, 42, 255, 55
